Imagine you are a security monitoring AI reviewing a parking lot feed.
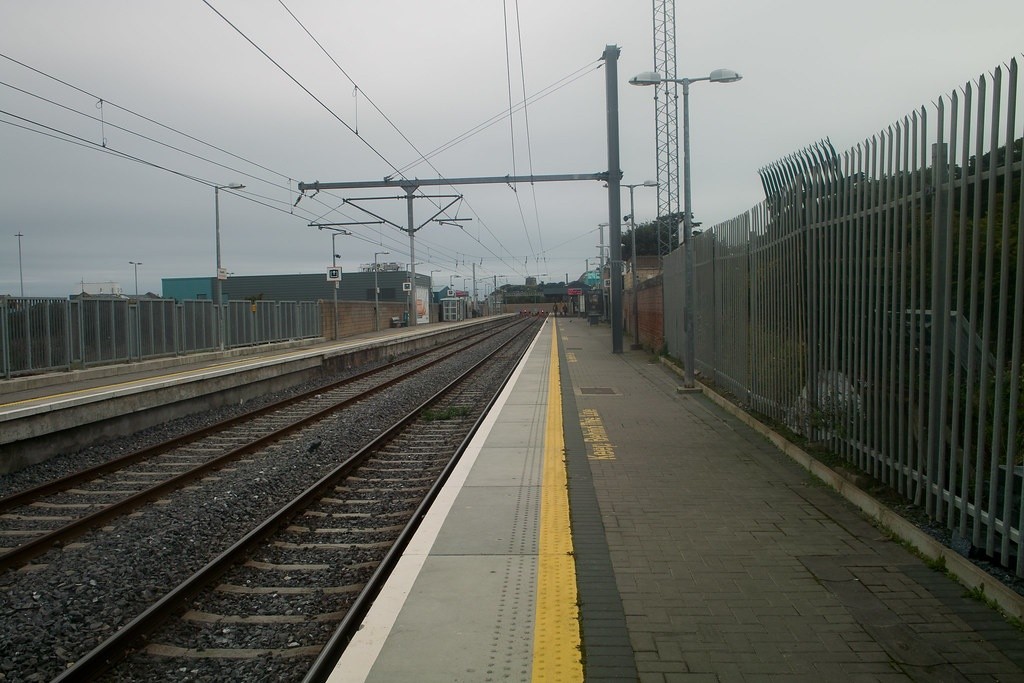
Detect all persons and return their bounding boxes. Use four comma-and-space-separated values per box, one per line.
552, 301, 558, 316
562, 304, 567, 316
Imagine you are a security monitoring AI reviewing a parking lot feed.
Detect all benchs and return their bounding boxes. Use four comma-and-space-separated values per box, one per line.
391, 317, 406, 327
